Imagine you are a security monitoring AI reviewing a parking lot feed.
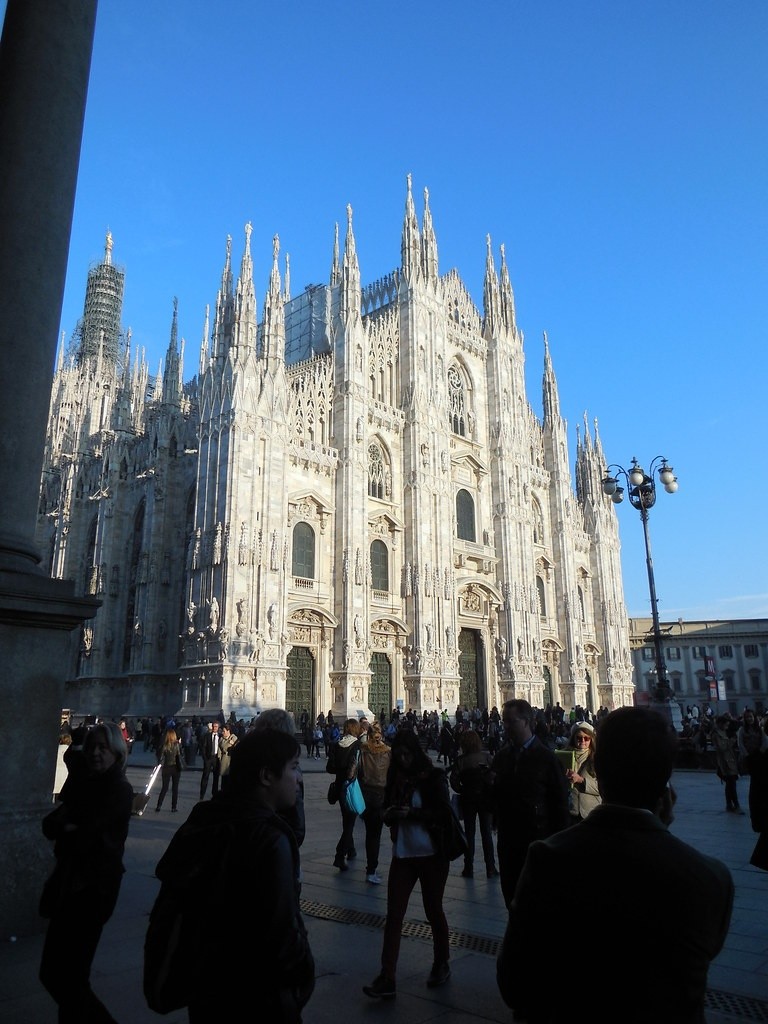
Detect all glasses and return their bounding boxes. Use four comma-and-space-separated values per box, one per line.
576, 736, 590, 741
499, 718, 520, 725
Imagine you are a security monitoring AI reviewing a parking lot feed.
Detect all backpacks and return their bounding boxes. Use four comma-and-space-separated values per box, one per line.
144, 801, 300, 1015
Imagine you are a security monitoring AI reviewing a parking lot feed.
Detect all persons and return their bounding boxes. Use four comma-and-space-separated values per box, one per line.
498, 706, 735, 1024
301, 702, 610, 998
659, 704, 768, 871
39, 710, 317, 1024
206, 597, 219, 633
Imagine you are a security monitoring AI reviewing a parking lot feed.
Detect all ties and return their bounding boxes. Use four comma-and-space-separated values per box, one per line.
212, 734, 217, 755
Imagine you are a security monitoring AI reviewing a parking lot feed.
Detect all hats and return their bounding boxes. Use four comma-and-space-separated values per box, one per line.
572, 721, 594, 732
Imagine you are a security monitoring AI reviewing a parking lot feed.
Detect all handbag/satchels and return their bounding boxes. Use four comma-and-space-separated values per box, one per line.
343, 750, 366, 815
176, 743, 188, 771
328, 782, 338, 805
445, 808, 469, 861
317, 741, 325, 747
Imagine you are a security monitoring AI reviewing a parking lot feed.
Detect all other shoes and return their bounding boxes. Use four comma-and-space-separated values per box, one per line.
346, 852, 357, 861
172, 809, 178, 812
733, 808, 745, 815
333, 859, 349, 871
487, 869, 500, 878
464, 867, 474, 878
727, 804, 734, 811
436, 759, 443, 763
366, 871, 381, 883
156, 807, 160, 812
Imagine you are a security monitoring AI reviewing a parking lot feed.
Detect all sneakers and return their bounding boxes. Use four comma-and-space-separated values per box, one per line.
428, 961, 450, 986
363, 975, 396, 998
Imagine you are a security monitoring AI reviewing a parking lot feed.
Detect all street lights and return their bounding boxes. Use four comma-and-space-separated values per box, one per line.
599, 454, 680, 703
705, 671, 723, 716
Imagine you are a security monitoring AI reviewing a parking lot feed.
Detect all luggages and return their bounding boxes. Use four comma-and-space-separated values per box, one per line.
130, 763, 162, 816
